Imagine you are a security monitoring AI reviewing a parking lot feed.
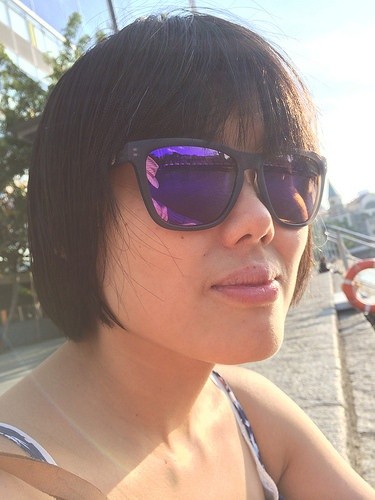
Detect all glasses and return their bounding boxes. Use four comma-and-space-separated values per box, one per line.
111, 137, 327, 231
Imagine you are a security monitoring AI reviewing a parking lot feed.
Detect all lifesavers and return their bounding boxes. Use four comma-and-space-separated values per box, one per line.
343, 261, 375, 313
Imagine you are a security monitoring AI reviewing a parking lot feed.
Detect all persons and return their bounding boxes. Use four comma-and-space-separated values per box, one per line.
1, 13, 374, 499
